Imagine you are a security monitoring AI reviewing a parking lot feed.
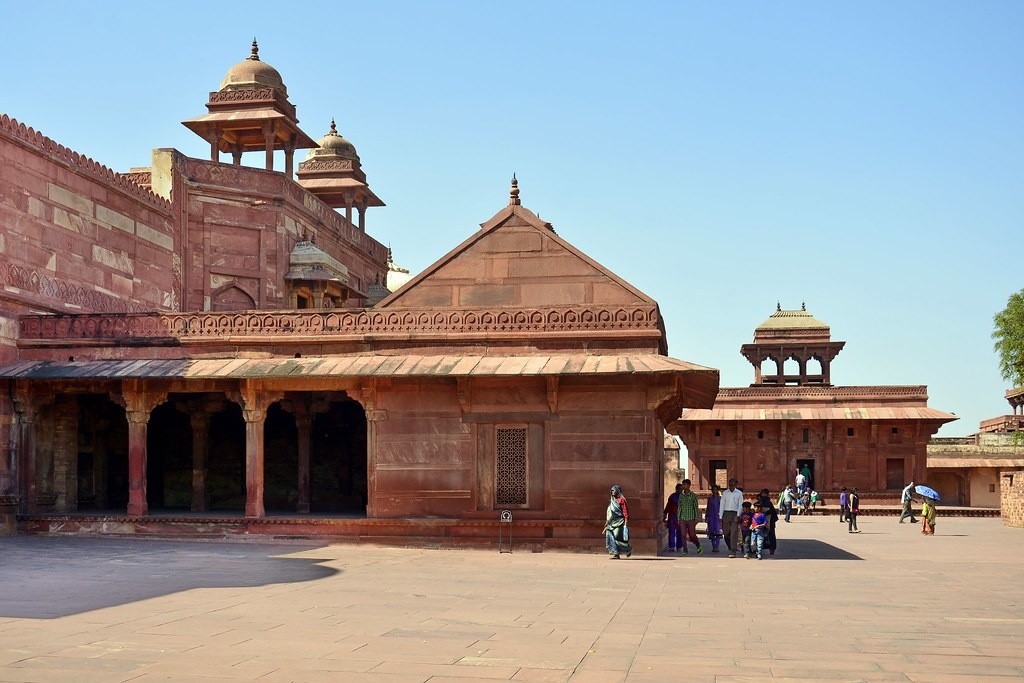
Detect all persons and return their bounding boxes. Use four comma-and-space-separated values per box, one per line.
777, 464, 821, 523
899, 482, 920, 523
603, 485, 633, 559
677, 479, 703, 557
663, 484, 683, 551
920, 496, 936, 535
705, 478, 778, 560
839, 487, 861, 533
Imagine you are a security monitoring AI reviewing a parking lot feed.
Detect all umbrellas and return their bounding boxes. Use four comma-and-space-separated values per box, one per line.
915, 485, 940, 501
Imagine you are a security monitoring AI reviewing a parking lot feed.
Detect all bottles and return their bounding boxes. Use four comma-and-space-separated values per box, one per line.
623, 525, 629, 541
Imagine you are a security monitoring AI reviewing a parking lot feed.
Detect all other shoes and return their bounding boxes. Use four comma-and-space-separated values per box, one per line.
670, 545, 774, 560
849, 530, 855, 534
855, 530, 862, 533
610, 555, 620, 559
626, 550, 631, 557
